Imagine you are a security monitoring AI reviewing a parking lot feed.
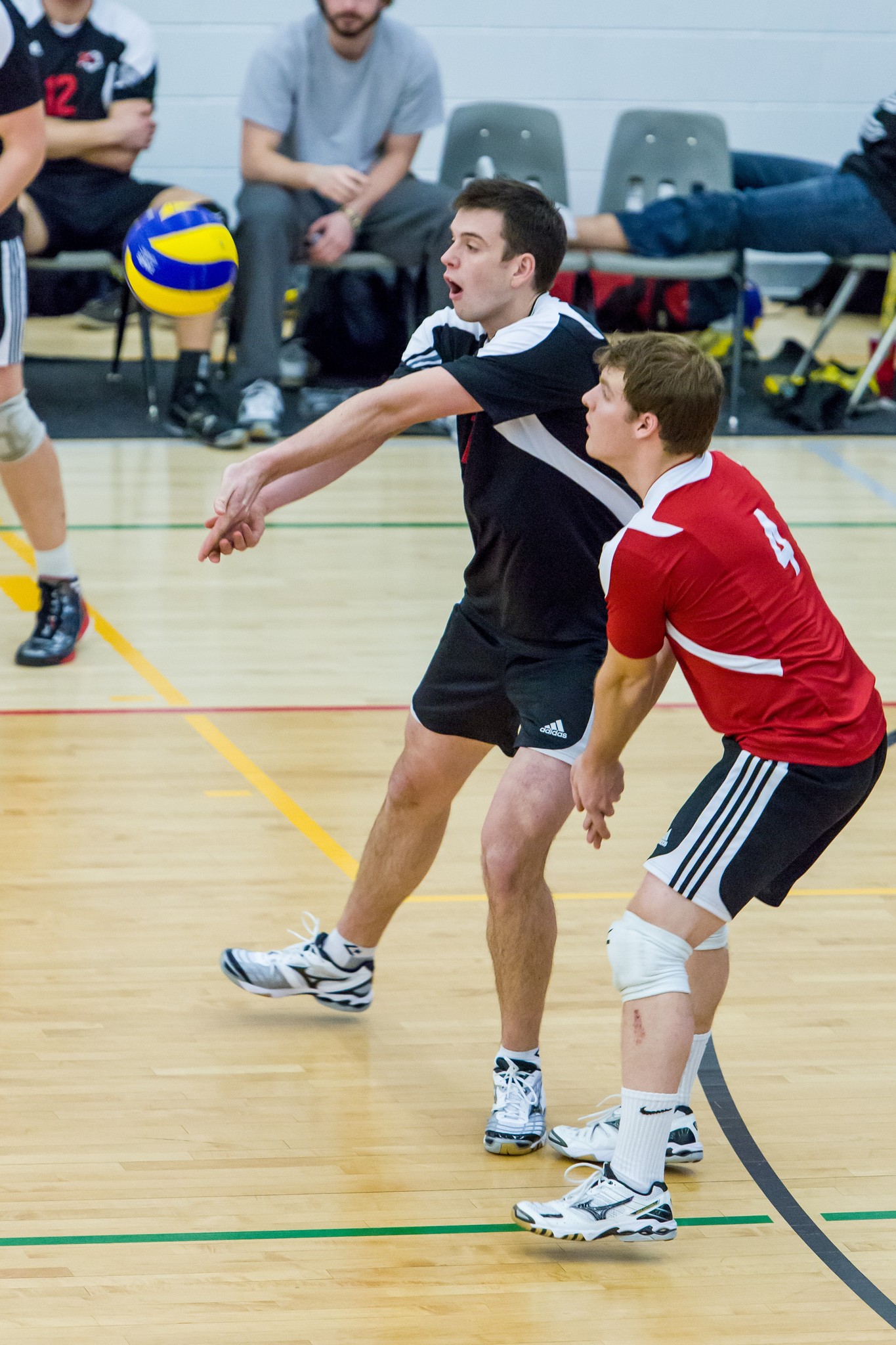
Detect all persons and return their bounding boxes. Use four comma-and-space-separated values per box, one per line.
0, 0, 82, 668
232, 1, 459, 444
199, 181, 643, 1160
476, 91, 896, 260
511, 332, 889, 1243
10, 0, 251, 451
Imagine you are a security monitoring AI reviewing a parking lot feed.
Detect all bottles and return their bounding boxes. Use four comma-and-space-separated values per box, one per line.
297, 387, 365, 415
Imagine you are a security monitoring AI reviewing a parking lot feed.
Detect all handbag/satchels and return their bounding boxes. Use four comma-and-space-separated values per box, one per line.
294, 266, 408, 390
598, 270, 737, 334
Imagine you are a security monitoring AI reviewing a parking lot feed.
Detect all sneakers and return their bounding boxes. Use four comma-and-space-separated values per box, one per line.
548, 1094, 703, 1163
511, 1159, 677, 1242
15, 581, 90, 666
482, 1057, 548, 1156
219, 911, 374, 1012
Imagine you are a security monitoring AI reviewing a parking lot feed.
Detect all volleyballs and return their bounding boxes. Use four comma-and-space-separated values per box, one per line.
121, 200, 240, 321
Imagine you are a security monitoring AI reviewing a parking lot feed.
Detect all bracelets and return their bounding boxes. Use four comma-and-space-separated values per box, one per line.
340, 204, 360, 234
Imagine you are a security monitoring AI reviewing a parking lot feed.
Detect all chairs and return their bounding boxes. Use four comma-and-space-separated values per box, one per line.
23, 101, 896, 432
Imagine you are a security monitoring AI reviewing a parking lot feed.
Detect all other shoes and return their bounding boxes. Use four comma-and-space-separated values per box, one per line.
164, 381, 249, 447
463, 154, 575, 242
237, 379, 285, 441
72, 292, 141, 328
